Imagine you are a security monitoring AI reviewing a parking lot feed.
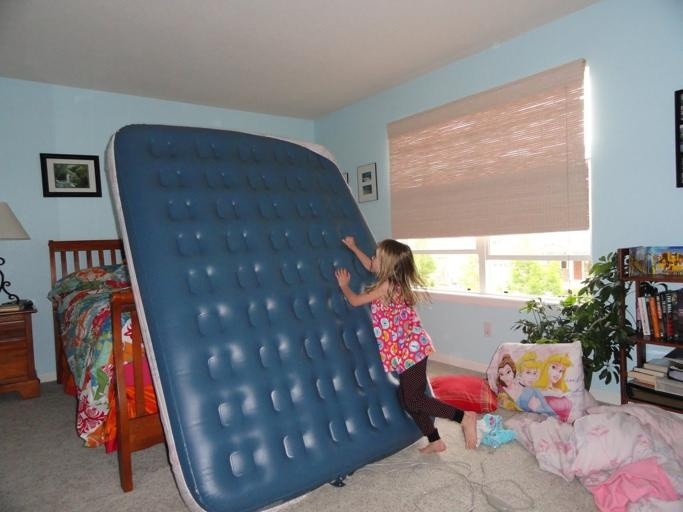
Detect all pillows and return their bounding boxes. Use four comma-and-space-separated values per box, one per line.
428, 342, 588, 420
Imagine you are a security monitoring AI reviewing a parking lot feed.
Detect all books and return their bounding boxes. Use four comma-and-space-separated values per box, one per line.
0, 299, 34, 311
628, 246, 683, 396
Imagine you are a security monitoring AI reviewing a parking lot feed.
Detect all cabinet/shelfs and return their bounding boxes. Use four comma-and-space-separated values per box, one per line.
618, 247, 683, 413
0, 308, 41, 399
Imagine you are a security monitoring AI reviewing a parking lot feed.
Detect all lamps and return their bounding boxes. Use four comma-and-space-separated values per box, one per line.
0, 201, 30, 306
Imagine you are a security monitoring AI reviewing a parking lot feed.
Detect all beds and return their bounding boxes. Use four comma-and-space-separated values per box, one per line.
48, 239, 166, 493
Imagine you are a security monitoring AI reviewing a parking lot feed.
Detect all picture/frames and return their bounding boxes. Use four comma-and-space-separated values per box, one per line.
39, 152, 102, 197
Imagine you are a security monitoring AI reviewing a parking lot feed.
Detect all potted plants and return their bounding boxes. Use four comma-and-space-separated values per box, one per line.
510, 250, 636, 395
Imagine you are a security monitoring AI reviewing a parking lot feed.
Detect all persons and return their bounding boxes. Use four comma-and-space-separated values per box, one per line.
497, 352, 572, 419
335, 236, 477, 453
